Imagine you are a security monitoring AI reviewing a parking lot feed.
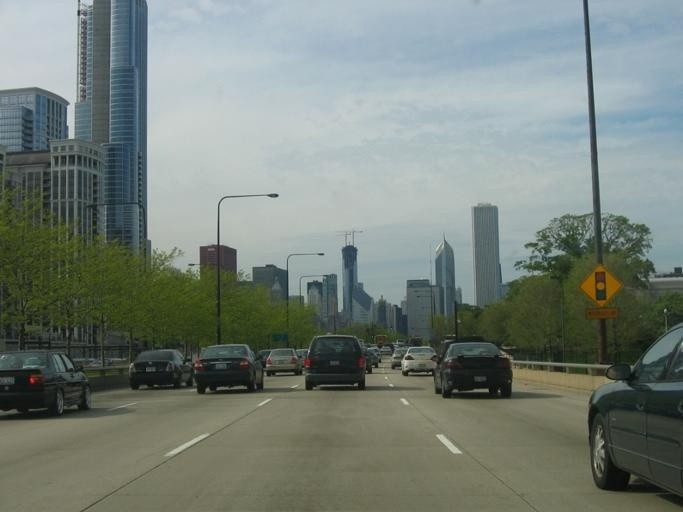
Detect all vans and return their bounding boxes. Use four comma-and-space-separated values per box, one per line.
302, 334, 367, 390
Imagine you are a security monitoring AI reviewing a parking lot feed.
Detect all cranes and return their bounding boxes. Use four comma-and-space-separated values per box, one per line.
335, 232, 351, 245
335, 229, 363, 247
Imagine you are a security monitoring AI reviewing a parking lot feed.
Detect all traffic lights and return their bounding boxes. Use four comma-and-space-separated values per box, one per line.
593, 270, 606, 302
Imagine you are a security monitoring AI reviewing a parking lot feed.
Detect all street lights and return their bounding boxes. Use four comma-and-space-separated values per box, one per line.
283, 273, 330, 346
411, 285, 436, 330
662, 307, 668, 333
186, 260, 225, 270
547, 273, 564, 374
262, 251, 326, 347
185, 191, 279, 345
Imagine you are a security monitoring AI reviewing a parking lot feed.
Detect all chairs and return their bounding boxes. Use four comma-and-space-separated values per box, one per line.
319, 345, 334, 351
341, 346, 352, 351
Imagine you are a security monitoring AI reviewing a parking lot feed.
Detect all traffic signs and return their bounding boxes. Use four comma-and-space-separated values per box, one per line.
584, 308, 618, 320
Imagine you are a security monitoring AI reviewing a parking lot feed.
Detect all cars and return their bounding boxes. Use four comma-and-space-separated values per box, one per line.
191, 343, 266, 395
258, 337, 485, 378
22, 355, 127, 371
583, 316, 682, 503
126, 348, 195, 391
0, 348, 95, 417
431, 336, 515, 399
